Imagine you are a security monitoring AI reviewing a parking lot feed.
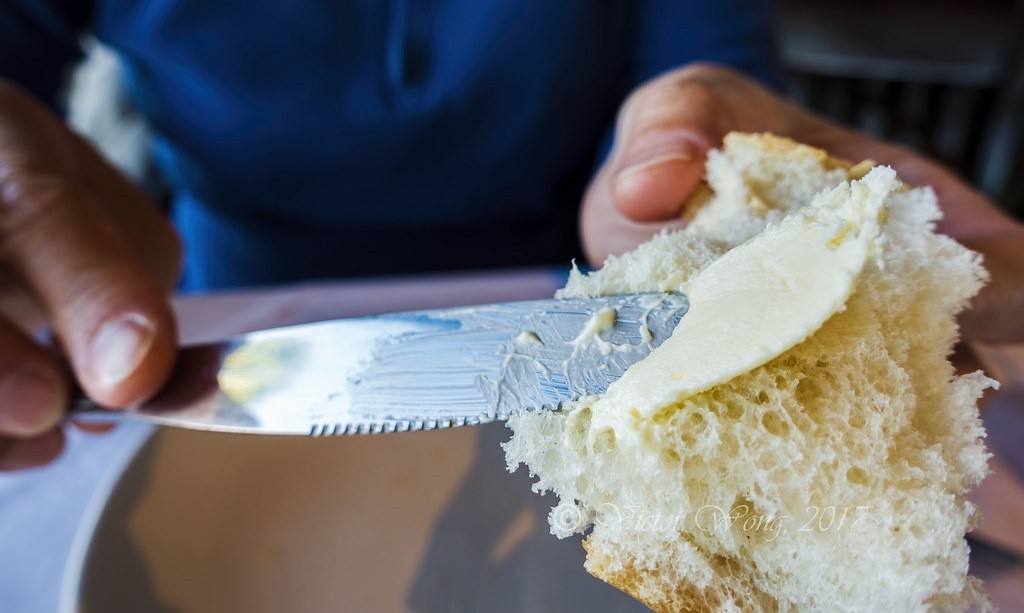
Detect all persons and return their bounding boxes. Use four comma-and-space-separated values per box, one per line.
0, 1, 1023, 476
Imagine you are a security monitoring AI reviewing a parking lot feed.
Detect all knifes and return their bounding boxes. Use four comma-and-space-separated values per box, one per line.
68, 290, 691, 437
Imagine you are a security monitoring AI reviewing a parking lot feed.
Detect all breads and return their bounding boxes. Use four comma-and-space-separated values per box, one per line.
499, 122, 997, 613
